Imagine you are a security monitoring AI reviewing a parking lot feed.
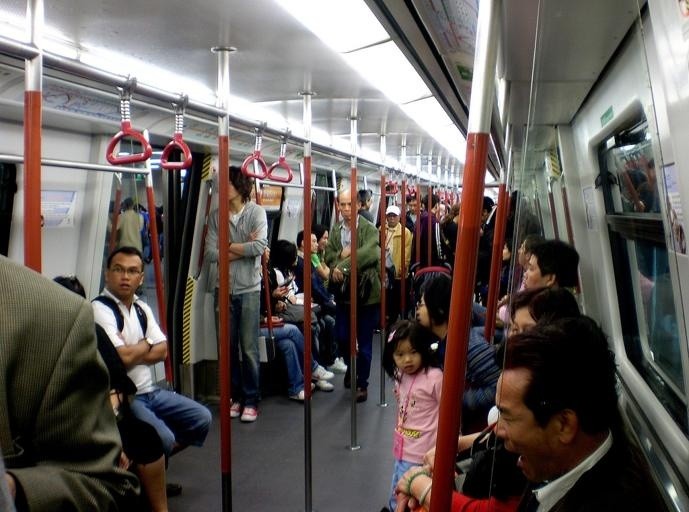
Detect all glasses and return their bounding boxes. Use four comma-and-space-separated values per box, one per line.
106, 267, 143, 276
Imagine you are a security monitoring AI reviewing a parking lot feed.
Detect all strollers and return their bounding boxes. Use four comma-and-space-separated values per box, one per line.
408, 261, 453, 318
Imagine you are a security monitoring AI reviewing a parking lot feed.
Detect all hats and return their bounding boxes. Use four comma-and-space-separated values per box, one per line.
385, 205, 401, 217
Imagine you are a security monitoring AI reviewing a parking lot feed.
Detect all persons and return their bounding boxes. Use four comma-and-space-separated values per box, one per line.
1, 256, 143, 512
87, 246, 212, 512
105, 197, 166, 294
204, 168, 269, 423
53, 278, 171, 512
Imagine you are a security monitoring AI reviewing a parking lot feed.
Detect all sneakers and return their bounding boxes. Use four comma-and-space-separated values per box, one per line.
240, 406, 259, 423
290, 356, 368, 404
229, 401, 242, 419
